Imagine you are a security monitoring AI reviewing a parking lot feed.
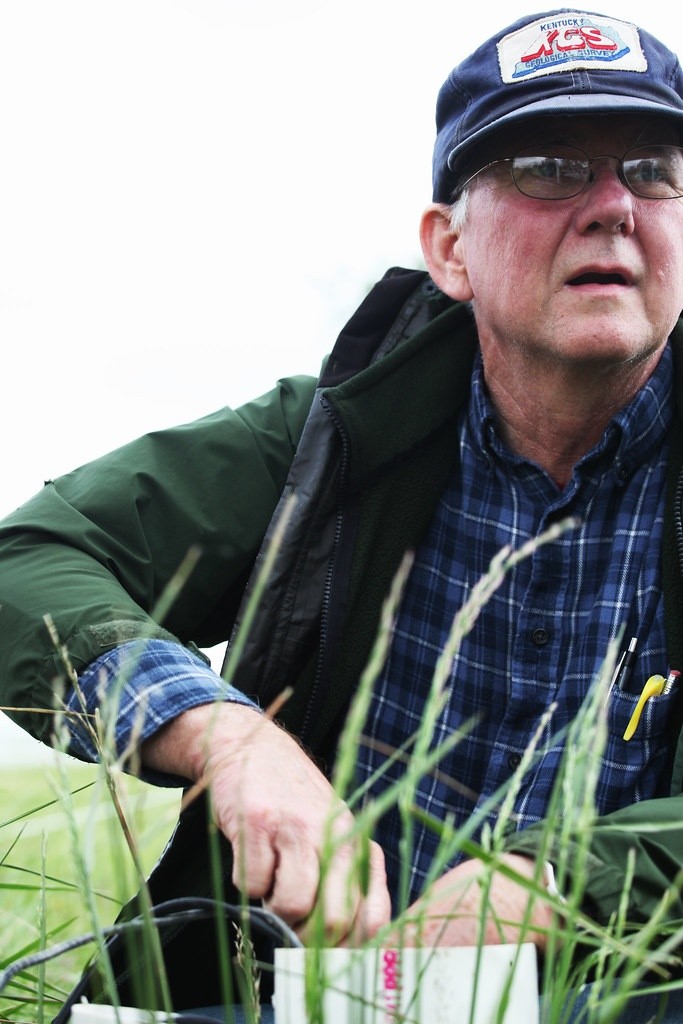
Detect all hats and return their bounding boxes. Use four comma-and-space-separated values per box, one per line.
432, 8, 683, 203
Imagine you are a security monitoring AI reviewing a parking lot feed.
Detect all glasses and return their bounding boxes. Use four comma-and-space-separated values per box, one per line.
454, 145, 683, 200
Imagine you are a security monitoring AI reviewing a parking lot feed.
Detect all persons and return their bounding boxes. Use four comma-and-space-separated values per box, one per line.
0, 7, 683, 1024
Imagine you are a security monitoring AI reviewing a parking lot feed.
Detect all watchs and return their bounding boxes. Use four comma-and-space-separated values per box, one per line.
545, 860, 574, 904
